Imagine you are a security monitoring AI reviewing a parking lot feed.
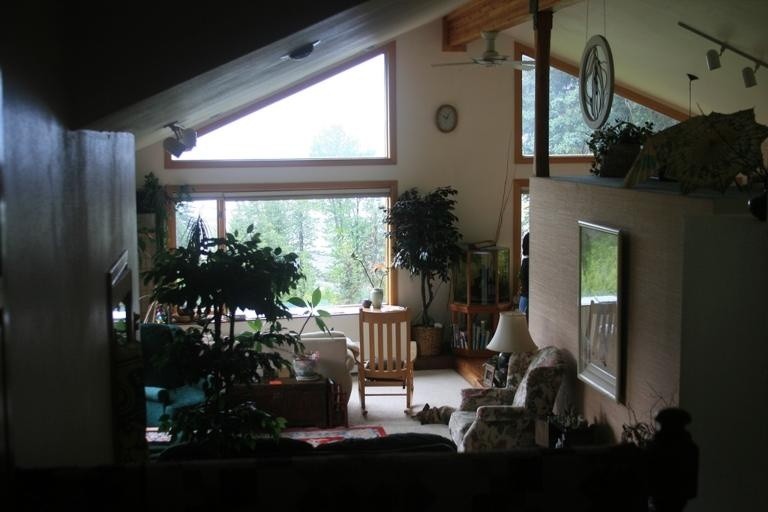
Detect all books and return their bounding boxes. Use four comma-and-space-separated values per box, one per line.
452, 319, 490, 351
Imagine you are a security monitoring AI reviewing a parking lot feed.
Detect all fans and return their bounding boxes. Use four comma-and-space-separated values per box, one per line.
429, 31, 535, 71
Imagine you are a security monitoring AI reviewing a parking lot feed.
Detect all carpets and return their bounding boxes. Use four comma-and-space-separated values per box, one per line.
145, 426, 386, 447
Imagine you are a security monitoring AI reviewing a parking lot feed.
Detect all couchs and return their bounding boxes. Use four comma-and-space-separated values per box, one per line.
202, 330, 360, 405
139, 323, 215, 426
1, 409, 698, 512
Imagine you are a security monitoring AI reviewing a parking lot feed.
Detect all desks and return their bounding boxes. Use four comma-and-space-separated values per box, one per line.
232, 375, 348, 429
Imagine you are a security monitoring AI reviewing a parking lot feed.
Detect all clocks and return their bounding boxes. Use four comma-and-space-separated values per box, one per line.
435, 104, 457, 133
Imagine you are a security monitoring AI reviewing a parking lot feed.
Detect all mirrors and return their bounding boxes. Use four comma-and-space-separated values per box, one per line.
576, 219, 622, 405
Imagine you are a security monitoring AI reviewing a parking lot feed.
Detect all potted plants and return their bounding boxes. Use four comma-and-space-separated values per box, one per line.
349, 251, 388, 309
379, 184, 464, 356
584, 118, 661, 178
178, 223, 306, 383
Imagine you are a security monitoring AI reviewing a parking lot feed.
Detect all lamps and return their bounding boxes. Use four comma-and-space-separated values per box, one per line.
163, 120, 197, 158
705, 46, 761, 88
485, 312, 538, 353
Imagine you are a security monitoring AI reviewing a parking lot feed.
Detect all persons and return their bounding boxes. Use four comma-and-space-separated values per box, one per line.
520, 233, 528, 324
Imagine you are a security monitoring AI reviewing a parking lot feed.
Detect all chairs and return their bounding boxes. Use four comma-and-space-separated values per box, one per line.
358, 307, 417, 410
447, 346, 577, 451
584, 299, 618, 363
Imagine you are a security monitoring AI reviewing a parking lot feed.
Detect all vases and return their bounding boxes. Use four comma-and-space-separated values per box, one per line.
292, 360, 313, 377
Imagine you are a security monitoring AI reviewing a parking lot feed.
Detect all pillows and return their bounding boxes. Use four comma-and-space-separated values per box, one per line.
158, 434, 458, 460
